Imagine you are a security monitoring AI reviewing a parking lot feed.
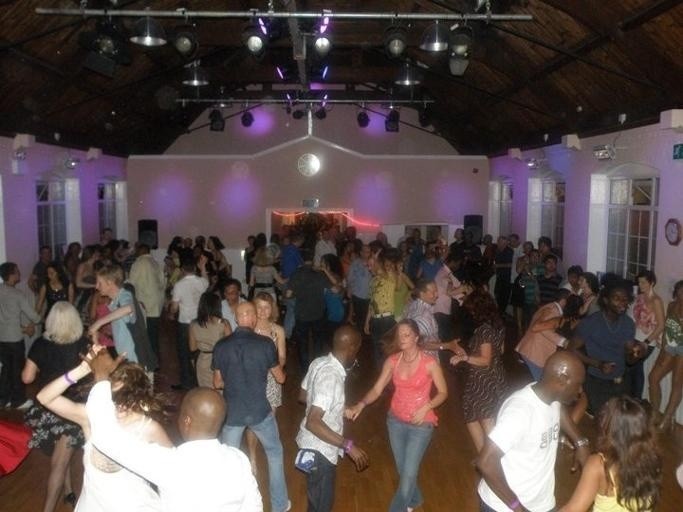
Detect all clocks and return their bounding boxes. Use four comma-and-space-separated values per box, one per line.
665, 219, 681, 244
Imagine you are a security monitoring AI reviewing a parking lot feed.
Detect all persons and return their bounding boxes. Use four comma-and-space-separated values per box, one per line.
78, 344, 263, 512
21, 301, 93, 511
477, 352, 591, 512
517, 264, 683, 450
558, 396, 663, 512
210, 303, 291, 512
36, 345, 173, 511
298, 327, 370, 512
351, 319, 447, 512
244, 229, 565, 467
1, 236, 286, 418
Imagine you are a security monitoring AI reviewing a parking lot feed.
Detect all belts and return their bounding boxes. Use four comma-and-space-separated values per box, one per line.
372, 311, 392, 318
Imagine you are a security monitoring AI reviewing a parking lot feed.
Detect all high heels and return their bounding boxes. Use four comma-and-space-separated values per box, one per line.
64, 492, 75, 508
652, 411, 676, 434
559, 435, 574, 450
345, 359, 359, 370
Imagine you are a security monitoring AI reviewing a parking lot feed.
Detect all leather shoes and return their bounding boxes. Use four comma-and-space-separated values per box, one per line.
172, 383, 183, 390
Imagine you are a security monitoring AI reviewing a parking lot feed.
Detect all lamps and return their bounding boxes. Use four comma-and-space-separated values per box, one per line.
591, 115, 627, 159
84, 10, 476, 87
523, 134, 550, 170
207, 97, 431, 131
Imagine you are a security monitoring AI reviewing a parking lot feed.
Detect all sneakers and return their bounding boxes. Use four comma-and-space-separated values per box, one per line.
16, 399, 34, 409
6, 402, 11, 407
285, 499, 291, 512
